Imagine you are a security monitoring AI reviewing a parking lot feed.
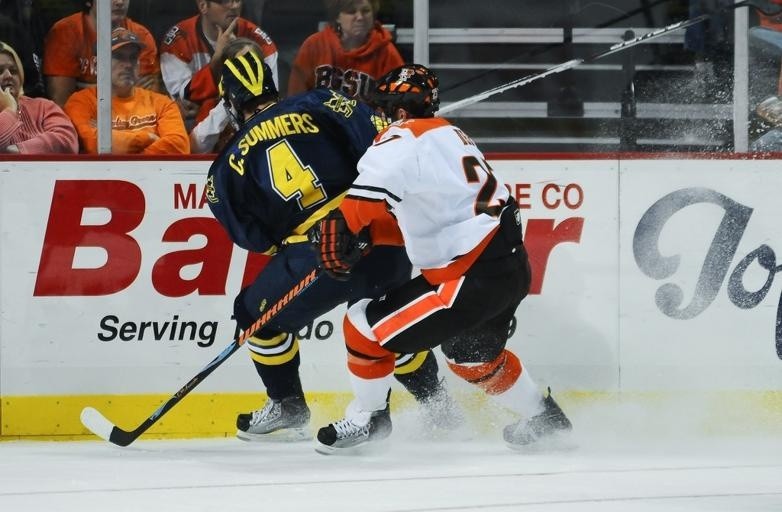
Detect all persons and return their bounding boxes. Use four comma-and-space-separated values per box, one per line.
41, 0, 160, 107
308, 66, 579, 458
186, 37, 266, 154
1, 41, 81, 154
206, 53, 469, 445
287, 0, 405, 98
159, 2, 278, 113
64, 32, 191, 156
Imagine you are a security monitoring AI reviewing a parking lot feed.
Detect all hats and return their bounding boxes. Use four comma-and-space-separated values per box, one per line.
111, 27, 147, 53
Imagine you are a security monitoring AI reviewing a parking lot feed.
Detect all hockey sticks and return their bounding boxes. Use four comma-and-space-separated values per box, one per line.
79, 241, 367, 448
434, 2, 781, 118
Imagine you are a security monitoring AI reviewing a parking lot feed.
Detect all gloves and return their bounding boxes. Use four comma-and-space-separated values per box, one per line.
317, 217, 371, 282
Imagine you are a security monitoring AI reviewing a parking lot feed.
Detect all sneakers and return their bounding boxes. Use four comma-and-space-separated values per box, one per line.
317, 404, 393, 449
503, 387, 570, 445
237, 395, 310, 434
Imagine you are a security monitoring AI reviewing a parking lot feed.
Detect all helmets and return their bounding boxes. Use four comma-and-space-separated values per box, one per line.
364, 64, 439, 116
220, 51, 278, 109
